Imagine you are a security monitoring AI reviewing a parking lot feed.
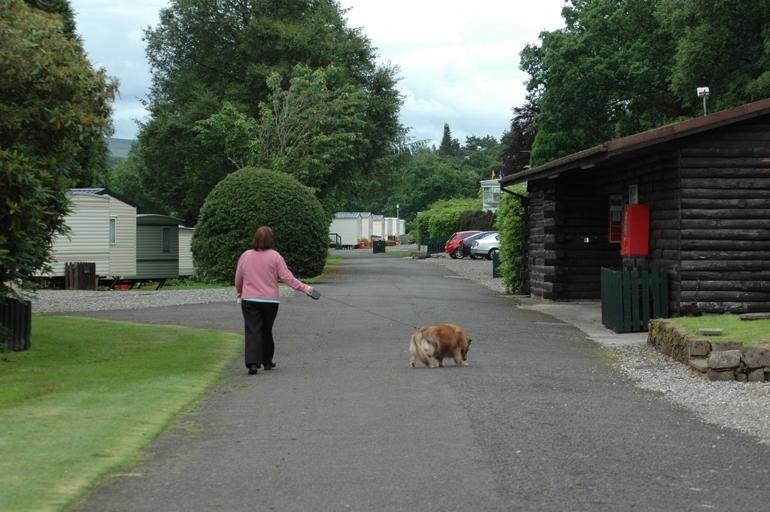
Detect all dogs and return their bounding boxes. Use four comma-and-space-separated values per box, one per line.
408, 322, 474, 369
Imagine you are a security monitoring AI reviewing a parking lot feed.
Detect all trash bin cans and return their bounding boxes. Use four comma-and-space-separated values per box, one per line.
492, 252, 504, 278
600, 265, 669, 334
65, 262, 97, 291
373, 240, 386, 253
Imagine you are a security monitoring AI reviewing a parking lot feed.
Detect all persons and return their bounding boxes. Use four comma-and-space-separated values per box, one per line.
235, 226, 315, 374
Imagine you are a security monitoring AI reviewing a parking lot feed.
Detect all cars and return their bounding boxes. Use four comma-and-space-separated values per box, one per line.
444, 229, 503, 261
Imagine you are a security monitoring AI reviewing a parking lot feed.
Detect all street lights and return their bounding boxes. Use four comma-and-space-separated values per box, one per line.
395, 204, 402, 235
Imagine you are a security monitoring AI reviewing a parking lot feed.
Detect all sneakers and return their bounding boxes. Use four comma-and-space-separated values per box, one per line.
247, 364, 257, 375
264, 361, 276, 370
